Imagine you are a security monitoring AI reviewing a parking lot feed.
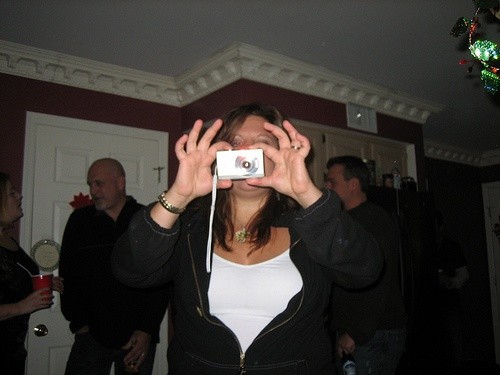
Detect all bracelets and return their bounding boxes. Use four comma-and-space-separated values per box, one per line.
158, 191, 186, 214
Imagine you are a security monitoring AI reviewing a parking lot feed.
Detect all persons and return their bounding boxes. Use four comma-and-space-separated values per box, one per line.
430, 209, 469, 364
0, 171, 63, 375
59, 159, 169, 375
111, 102, 383, 375
327, 155, 408, 375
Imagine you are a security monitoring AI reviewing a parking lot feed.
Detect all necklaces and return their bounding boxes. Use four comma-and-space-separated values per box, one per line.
234, 228, 247, 243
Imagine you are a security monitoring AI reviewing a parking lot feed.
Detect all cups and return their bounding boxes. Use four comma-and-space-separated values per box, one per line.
32, 274, 53, 302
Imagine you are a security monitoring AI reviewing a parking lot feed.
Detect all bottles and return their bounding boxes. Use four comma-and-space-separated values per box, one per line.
343, 350, 357, 375
391, 160, 400, 188
126, 339, 138, 373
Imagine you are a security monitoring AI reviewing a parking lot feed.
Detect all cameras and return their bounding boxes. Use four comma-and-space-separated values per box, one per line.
217, 149, 264, 180
